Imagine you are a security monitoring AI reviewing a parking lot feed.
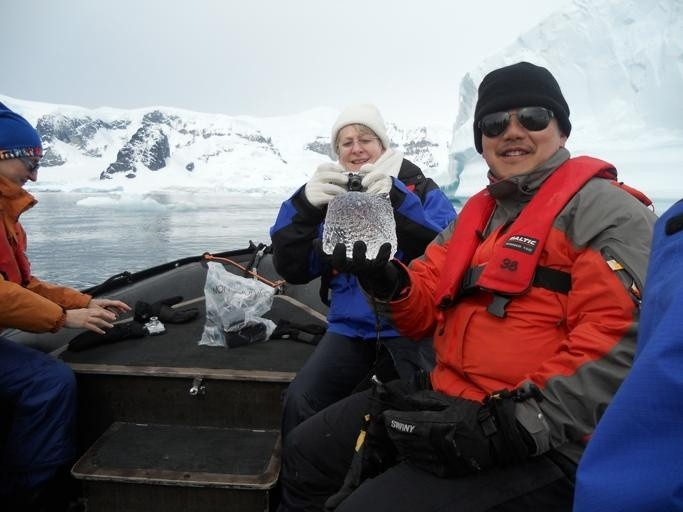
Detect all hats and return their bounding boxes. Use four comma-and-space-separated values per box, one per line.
331, 101, 389, 151
472, 62, 572, 153
0, 101, 42, 160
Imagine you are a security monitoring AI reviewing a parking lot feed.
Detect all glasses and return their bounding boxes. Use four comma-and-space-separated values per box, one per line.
19, 155, 40, 174
476, 105, 556, 139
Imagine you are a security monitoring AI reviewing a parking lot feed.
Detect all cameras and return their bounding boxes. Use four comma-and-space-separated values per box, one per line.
329, 172, 368, 193
144, 316, 167, 336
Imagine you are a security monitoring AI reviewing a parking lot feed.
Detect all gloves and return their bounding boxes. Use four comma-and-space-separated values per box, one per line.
380, 384, 551, 478
271, 317, 326, 344
67, 322, 146, 352
134, 296, 200, 324
315, 239, 408, 305
359, 163, 392, 197
304, 160, 351, 208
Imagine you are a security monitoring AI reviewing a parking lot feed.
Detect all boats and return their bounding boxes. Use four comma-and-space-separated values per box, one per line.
0, 235, 552, 512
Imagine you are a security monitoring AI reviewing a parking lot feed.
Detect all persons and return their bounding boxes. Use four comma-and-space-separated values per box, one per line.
270, 99, 460, 512
1, 103, 132, 509
570, 193, 683, 512
278, 63, 661, 509
